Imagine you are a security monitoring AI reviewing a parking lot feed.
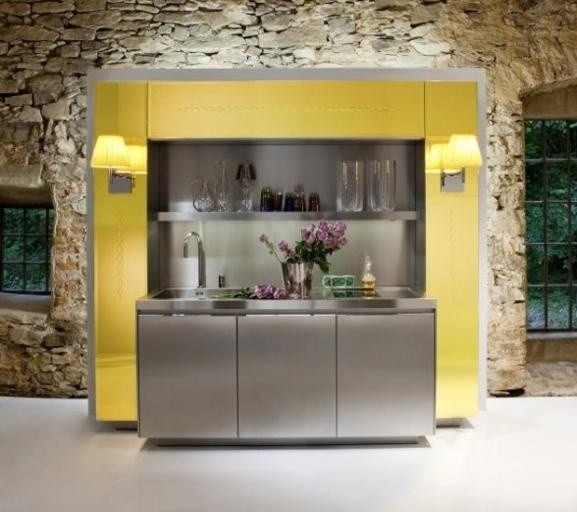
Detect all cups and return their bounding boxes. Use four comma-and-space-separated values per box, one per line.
336, 159, 397, 213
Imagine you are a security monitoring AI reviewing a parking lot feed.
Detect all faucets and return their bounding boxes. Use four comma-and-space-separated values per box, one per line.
182, 231, 207, 287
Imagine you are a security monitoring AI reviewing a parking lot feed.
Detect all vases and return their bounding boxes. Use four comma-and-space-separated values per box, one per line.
280, 260, 316, 300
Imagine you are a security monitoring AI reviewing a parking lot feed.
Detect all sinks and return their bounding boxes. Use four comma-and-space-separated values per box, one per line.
153, 288, 250, 302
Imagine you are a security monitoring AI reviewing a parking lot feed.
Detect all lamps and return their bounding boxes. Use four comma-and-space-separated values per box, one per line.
425, 135, 483, 191
90, 135, 148, 193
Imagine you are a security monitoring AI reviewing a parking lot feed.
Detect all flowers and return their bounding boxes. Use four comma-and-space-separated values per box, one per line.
210, 283, 289, 299
259, 221, 341, 286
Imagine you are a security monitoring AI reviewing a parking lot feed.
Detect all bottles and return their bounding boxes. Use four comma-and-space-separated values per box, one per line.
258, 185, 321, 213
193, 158, 233, 212
